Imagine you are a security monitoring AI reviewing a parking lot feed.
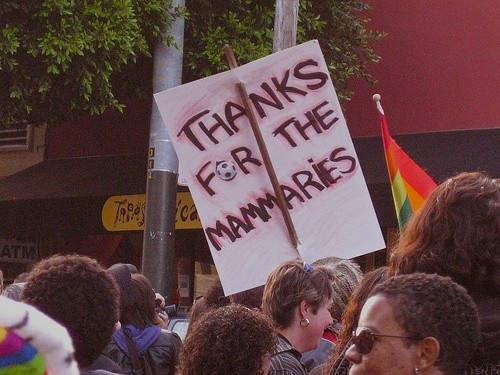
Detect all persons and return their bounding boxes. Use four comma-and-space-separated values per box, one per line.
186, 286, 230, 340
177, 305, 279, 375
345, 273, 481, 375
21, 255, 121, 375
14, 272, 29, 283
154, 293, 169, 329
104, 274, 182, 374
0, 295, 80, 375
262, 258, 333, 375
300, 257, 363, 372
3, 283, 25, 303
389, 171, 500, 375
309, 267, 390, 375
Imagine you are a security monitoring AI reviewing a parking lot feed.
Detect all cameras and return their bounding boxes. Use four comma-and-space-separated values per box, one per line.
148, 299, 164, 324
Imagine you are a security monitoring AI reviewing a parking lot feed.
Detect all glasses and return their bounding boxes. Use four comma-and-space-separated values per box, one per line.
346, 327, 421, 356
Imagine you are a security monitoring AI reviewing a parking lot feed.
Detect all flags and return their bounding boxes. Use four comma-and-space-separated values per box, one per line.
373, 94, 437, 233
